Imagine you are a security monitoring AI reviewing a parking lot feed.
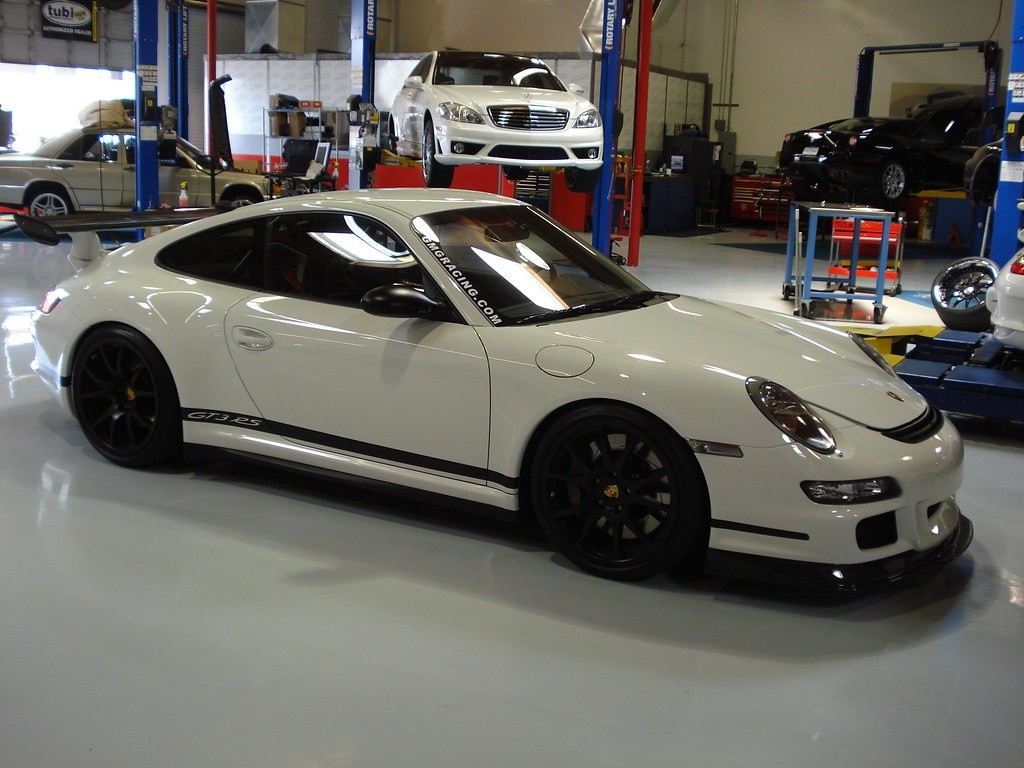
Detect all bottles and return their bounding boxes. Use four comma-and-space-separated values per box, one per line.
645, 160, 651, 175
179, 189, 188, 207
662, 163, 666, 175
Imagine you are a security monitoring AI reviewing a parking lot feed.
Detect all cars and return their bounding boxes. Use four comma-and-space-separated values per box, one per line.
386, 50, 605, 187
986, 248, 1024, 352
35, 182, 976, 595
0, 74, 272, 219
781, 94, 988, 208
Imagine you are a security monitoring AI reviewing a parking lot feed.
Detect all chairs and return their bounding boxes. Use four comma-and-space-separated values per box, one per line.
613, 157, 632, 234
482, 75, 501, 85
435, 73, 455, 85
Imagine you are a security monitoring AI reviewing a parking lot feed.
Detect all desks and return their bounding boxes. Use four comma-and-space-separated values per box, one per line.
614, 173, 707, 228
265, 175, 336, 199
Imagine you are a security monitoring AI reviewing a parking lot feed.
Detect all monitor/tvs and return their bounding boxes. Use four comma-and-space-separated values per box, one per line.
670, 154, 684, 170
314, 142, 332, 173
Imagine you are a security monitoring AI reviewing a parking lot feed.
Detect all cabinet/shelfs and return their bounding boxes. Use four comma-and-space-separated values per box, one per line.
262, 107, 350, 191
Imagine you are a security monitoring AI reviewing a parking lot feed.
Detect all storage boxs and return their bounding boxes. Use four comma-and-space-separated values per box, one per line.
269, 95, 280, 109
299, 101, 323, 109
268, 111, 287, 136
289, 112, 305, 137
322, 112, 349, 145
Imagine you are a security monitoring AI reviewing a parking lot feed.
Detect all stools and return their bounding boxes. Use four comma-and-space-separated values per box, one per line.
694, 198, 718, 227
